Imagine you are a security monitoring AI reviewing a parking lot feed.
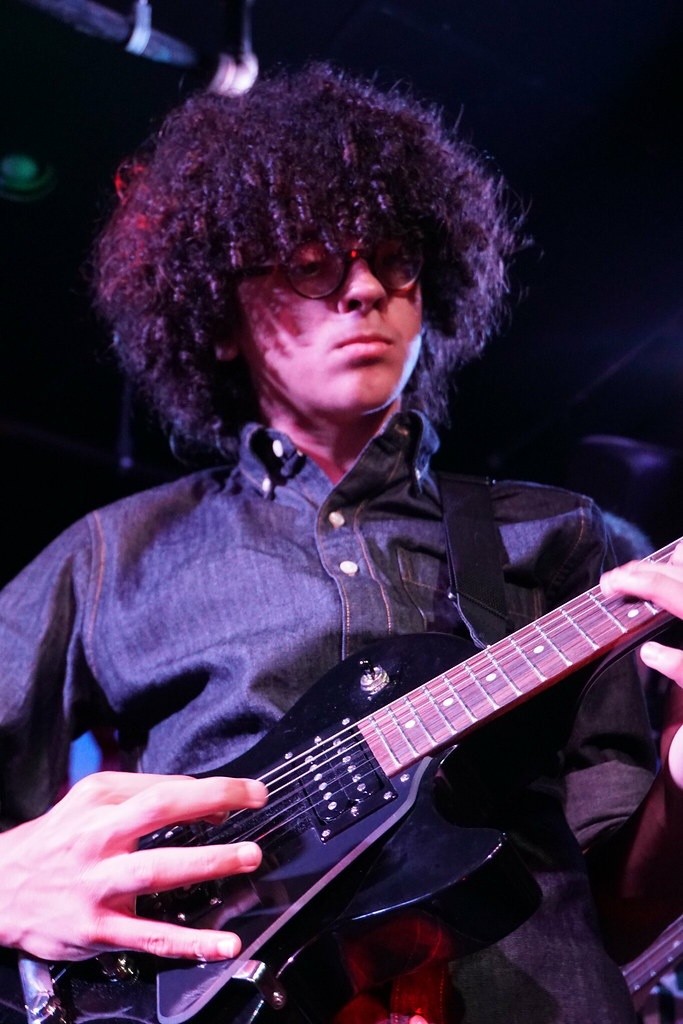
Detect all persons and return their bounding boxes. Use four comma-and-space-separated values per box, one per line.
1, 72, 683, 1024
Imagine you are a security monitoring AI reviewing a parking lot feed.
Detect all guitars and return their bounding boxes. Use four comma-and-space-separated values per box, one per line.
618, 915, 683, 997
0, 531, 683, 1024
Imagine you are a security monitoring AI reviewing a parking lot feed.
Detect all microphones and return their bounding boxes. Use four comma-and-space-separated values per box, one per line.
29, 0, 260, 96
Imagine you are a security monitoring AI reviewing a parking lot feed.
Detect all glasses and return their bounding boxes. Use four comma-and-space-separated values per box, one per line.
235, 238, 427, 299
